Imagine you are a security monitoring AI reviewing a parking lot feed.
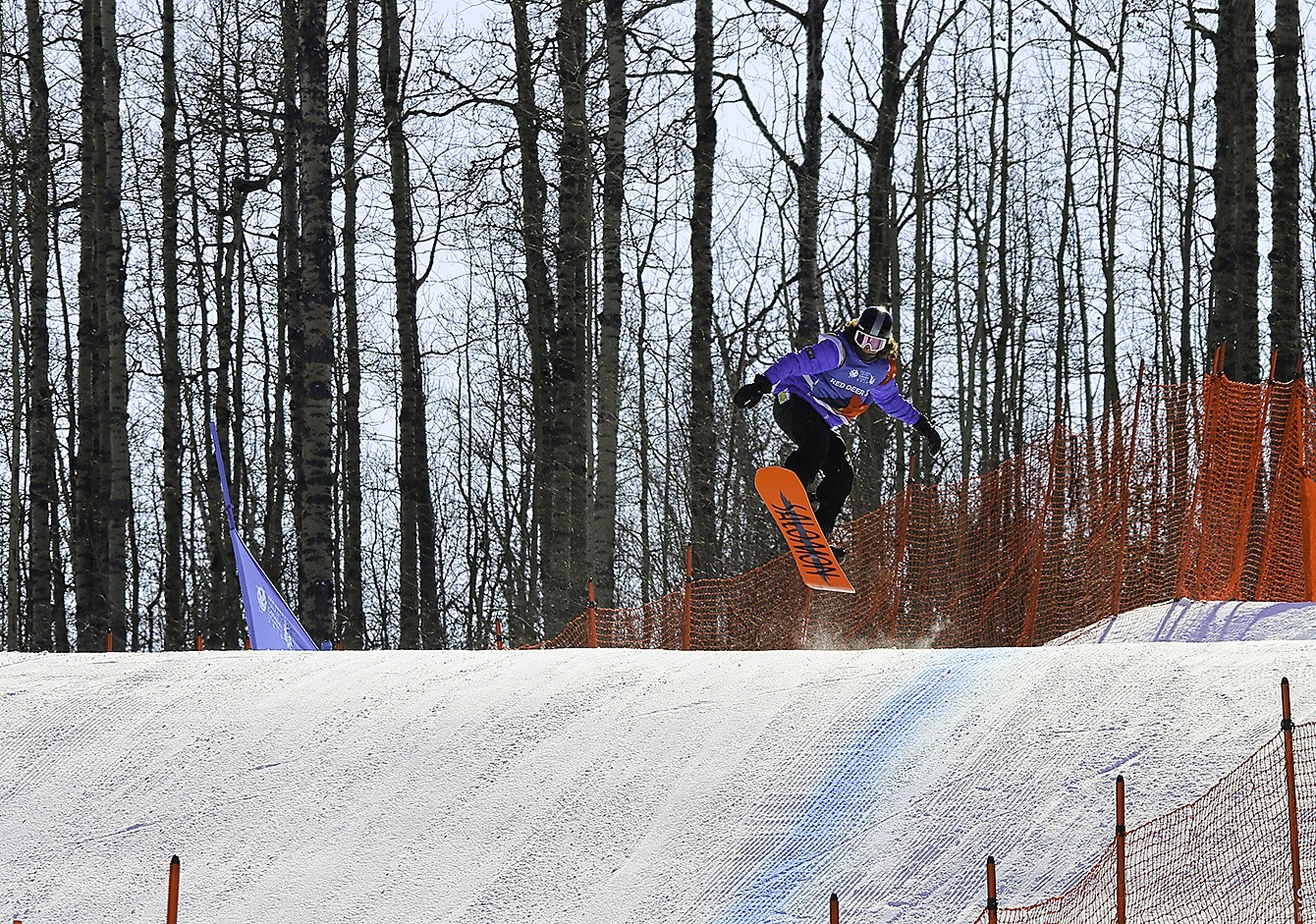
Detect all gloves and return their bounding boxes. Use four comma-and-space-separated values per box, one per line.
732, 373, 772, 409
912, 415, 941, 456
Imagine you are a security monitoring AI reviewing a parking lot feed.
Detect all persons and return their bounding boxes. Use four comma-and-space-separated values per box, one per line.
733, 305, 941, 566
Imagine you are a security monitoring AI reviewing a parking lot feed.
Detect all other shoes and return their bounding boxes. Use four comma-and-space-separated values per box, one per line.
830, 547, 845, 565
807, 492, 819, 512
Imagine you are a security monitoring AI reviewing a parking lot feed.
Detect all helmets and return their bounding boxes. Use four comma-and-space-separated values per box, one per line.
858, 306, 892, 338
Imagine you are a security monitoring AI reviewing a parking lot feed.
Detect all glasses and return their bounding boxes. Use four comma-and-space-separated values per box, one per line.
854, 330, 887, 351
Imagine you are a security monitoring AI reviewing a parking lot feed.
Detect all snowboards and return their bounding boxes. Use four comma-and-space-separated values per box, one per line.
754, 467, 856, 593
